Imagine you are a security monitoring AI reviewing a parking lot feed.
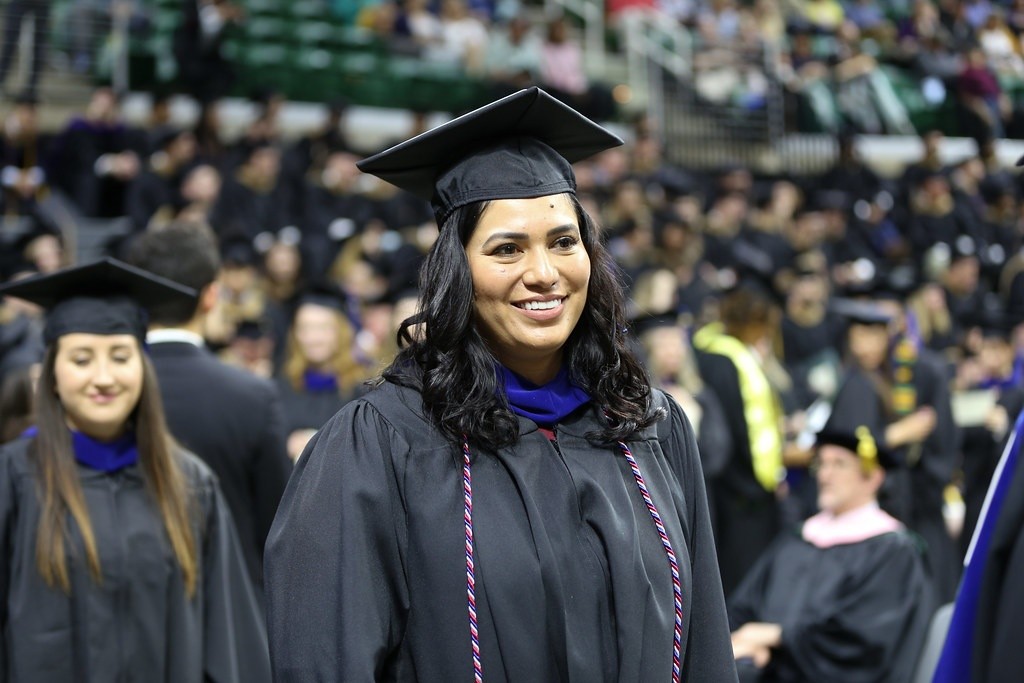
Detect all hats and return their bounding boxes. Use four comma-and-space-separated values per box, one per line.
811, 369, 902, 473
1, 259, 194, 331
356, 87, 623, 229
570, 156, 1024, 350
0, 75, 439, 318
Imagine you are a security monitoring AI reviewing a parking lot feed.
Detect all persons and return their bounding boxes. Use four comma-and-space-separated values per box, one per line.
0, 0, 1024, 606
0, 292, 277, 683
724, 414, 937, 682
259, 135, 739, 682
